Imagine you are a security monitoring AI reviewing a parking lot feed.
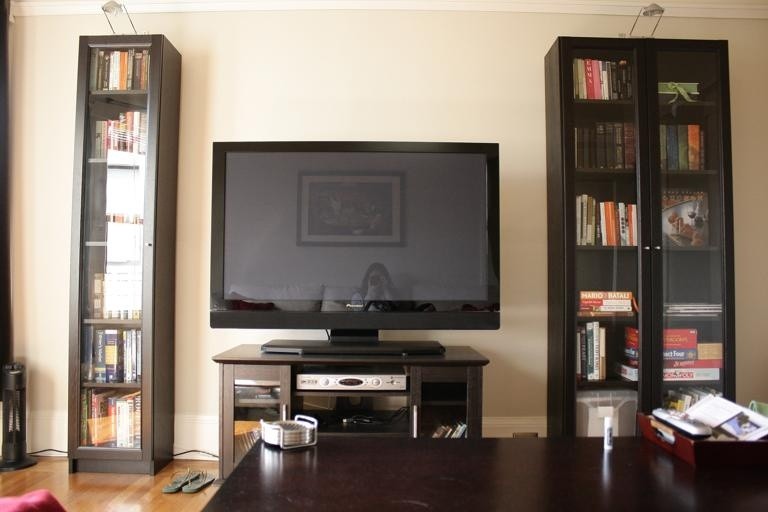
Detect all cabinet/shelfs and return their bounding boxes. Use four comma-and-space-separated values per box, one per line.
545, 36, 736, 438
210, 341, 489, 485
66, 35, 182, 477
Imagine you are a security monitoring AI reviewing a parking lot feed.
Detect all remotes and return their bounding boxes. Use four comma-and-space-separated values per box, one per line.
652, 408, 713, 438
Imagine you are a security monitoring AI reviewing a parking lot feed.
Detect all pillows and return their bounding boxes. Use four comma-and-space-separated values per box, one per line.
230, 290, 273, 310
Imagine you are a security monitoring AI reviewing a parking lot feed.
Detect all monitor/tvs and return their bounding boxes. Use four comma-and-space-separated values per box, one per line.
209, 140, 501, 355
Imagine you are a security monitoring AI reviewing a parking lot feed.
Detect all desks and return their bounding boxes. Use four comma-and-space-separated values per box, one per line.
200, 431, 768, 511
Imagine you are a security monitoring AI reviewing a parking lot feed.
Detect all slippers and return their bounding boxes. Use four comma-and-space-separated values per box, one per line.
161, 467, 202, 493
181, 469, 215, 493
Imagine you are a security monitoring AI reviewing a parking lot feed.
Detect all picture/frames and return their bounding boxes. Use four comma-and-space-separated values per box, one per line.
298, 170, 403, 247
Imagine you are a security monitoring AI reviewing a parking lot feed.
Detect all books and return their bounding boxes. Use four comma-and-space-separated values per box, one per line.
664, 385, 724, 412
660, 125, 705, 172
572, 57, 638, 172
233, 378, 281, 402
575, 291, 633, 381
77, 44, 154, 455
576, 194, 638, 248
613, 325, 724, 381
423, 419, 467, 438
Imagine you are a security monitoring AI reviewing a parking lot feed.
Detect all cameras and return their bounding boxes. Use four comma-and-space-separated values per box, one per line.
370, 275, 387, 287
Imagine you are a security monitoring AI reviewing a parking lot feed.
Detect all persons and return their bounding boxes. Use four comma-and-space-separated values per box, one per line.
349, 259, 395, 311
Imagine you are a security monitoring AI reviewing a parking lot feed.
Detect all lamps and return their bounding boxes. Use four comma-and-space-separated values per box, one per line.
630, 3, 664, 34
102, 0, 137, 34
